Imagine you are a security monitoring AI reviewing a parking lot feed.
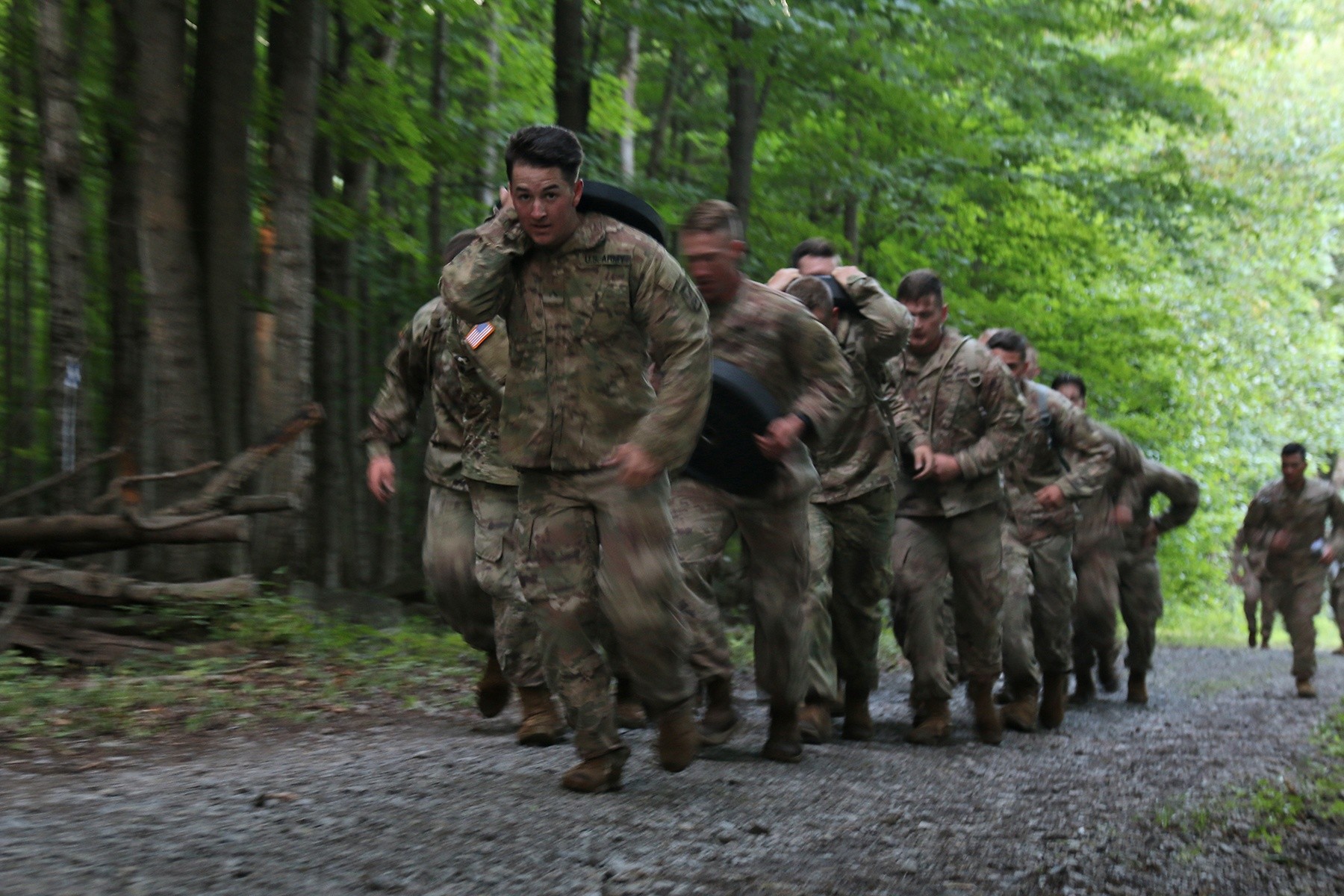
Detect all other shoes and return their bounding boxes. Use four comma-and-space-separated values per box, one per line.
1127, 673, 1147, 702
700, 711, 745, 745
1007, 690, 1045, 731
479, 652, 512, 719
1297, 678, 1317, 697
1044, 686, 1069, 730
1070, 676, 1095, 711
980, 704, 1006, 744
761, 719, 807, 764
802, 701, 832, 744
520, 700, 564, 743
908, 697, 953, 744
562, 747, 628, 793
841, 700, 875, 740
1097, 666, 1118, 692
655, 700, 699, 772
610, 697, 652, 727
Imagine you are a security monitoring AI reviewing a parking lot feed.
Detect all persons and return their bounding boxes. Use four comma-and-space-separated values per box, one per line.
789, 236, 934, 740
439, 127, 709, 795
436, 259, 649, 736
1228, 443, 1344, 700
664, 197, 857, 761
763, 257, 916, 745
368, 227, 478, 762
976, 327, 1205, 734
882, 271, 1027, 752
